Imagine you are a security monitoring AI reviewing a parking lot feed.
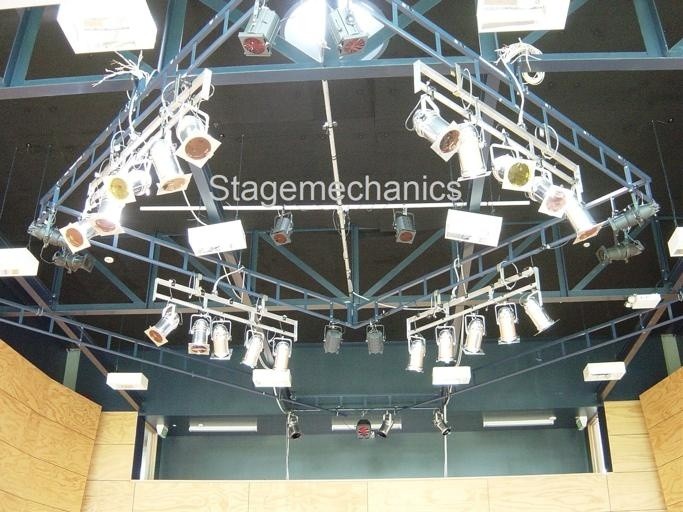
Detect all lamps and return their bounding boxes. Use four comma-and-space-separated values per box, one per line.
393, 211, 417, 245
413, 94, 601, 245
238, 7, 281, 56
366, 325, 385, 355
332, 420, 405, 430
325, 7, 369, 58
608, 202, 660, 233
406, 296, 557, 373
286, 416, 301, 440
597, 240, 645, 264
189, 422, 258, 432
60, 115, 222, 254
482, 418, 555, 429
434, 412, 451, 437
356, 420, 372, 440
271, 212, 294, 246
144, 312, 292, 373
323, 326, 343, 354
378, 413, 393, 438
